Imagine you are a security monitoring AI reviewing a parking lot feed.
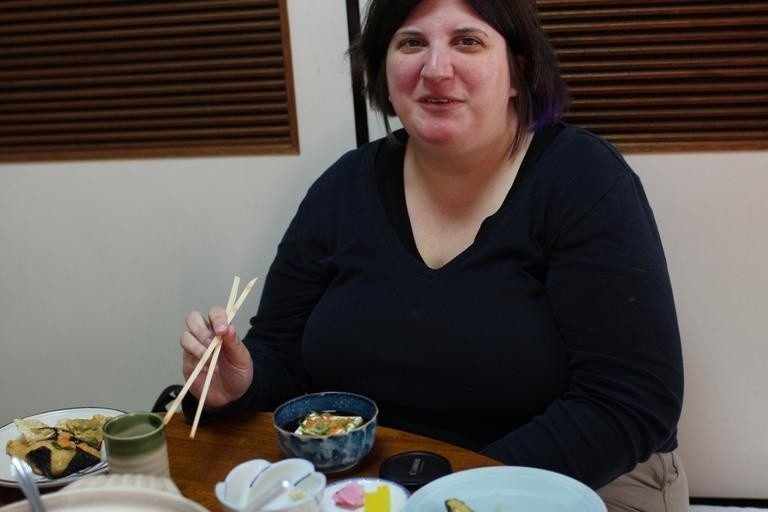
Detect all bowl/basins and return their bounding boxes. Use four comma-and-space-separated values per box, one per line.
271, 390, 380, 474
214, 472, 326, 511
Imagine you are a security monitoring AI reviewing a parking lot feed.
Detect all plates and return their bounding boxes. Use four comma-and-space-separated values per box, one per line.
322, 475, 414, 511
0, 406, 130, 490
402, 464, 609, 511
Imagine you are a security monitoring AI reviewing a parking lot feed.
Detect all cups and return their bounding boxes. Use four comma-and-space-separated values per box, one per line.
99, 411, 171, 480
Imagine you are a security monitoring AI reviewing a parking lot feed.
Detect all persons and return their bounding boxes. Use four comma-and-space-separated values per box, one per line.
176, 1, 695, 512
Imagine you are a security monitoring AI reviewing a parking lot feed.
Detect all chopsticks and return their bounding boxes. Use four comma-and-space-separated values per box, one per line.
163, 275, 260, 442
